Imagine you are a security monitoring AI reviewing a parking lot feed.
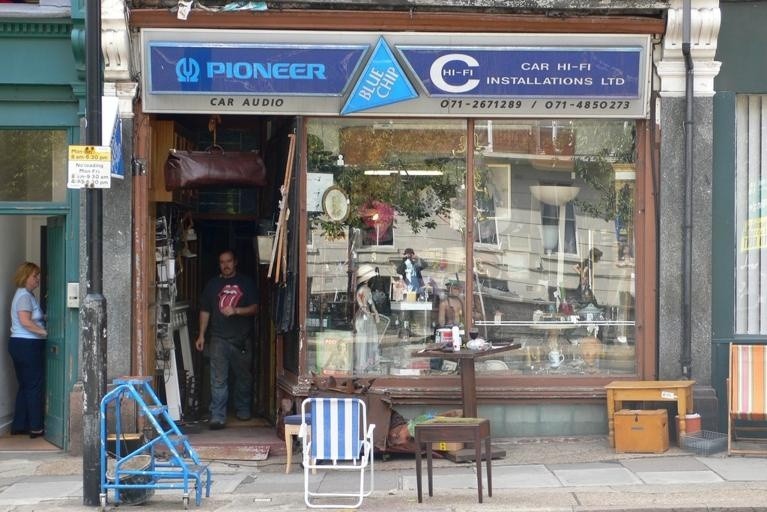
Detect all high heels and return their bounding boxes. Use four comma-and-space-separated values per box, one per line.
11, 430, 43, 438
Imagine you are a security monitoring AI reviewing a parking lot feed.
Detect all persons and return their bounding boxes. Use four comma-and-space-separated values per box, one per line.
573, 248, 603, 309
354, 264, 380, 372
8, 263, 48, 439
195, 249, 261, 430
397, 248, 428, 288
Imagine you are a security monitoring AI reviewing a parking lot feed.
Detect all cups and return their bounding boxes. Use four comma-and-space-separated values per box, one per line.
549, 352, 564, 369
469, 328, 478, 339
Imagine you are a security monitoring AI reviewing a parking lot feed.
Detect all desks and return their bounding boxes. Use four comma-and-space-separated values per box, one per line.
411, 344, 522, 462
604, 380, 696, 448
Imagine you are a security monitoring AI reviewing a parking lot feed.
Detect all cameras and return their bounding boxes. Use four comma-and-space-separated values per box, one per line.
405, 253, 413, 259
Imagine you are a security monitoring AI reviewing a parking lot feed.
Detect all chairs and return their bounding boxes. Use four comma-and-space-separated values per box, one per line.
725, 342, 767, 457
298, 396, 376, 509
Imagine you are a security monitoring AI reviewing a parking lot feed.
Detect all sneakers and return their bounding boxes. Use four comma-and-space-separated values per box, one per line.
208, 422, 225, 430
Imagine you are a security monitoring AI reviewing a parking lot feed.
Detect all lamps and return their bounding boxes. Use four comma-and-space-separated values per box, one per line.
528, 172, 582, 208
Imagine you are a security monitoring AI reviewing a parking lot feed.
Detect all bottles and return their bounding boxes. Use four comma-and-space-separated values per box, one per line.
451, 324, 461, 351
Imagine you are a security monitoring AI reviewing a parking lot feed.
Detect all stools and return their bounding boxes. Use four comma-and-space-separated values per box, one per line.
613, 408, 669, 454
415, 416, 492, 503
283, 413, 317, 475
